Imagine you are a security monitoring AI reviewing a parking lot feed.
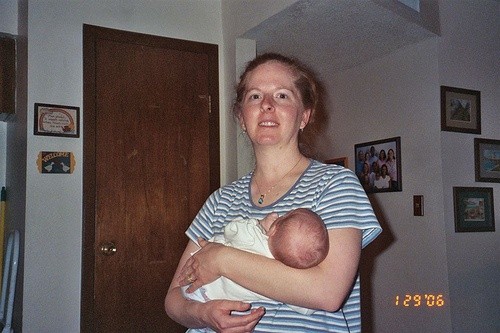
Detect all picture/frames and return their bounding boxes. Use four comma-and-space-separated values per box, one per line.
354, 136, 402, 194
453, 186, 496, 233
474, 137, 500, 183
440, 85, 481, 135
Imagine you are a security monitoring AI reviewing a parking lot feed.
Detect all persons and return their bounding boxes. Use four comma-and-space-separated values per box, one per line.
181, 208, 329, 303
164, 52, 382, 332
357, 146, 396, 190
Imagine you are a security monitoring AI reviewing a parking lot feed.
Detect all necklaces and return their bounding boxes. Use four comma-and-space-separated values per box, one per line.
253, 155, 305, 204
